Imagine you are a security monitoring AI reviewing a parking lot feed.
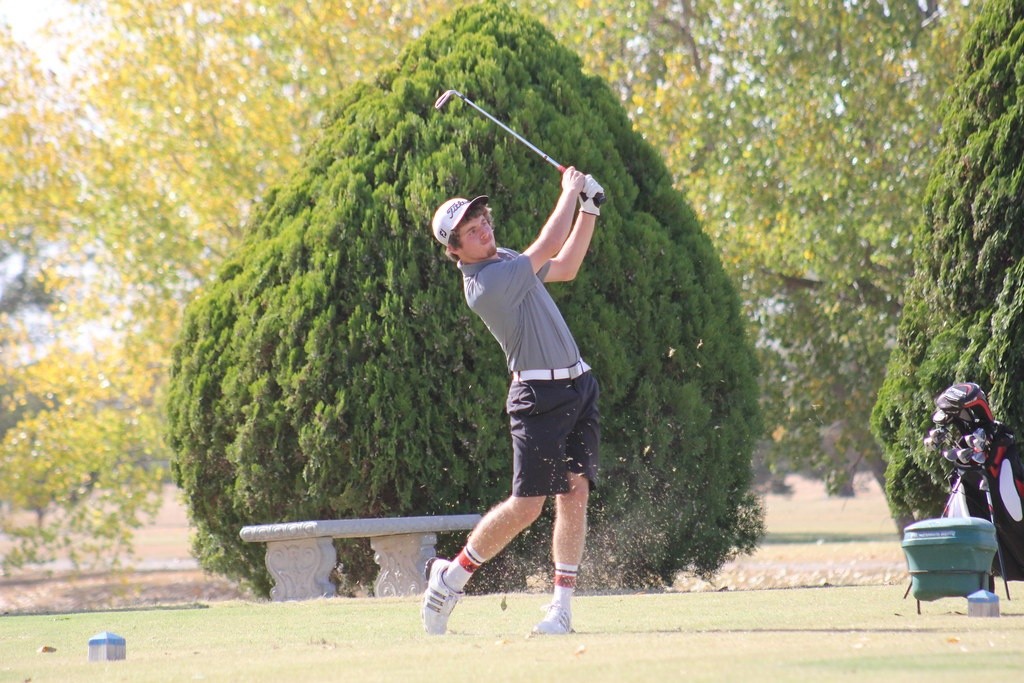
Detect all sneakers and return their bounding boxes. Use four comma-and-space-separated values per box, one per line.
534, 601, 572, 634
421, 557, 465, 635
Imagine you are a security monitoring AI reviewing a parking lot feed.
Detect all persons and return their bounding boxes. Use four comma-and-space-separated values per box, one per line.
422, 166, 605, 635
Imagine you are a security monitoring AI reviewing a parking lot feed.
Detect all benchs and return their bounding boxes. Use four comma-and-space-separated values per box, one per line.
240, 514, 481, 604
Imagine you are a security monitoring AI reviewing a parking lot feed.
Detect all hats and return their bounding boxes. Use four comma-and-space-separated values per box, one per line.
432, 195, 490, 246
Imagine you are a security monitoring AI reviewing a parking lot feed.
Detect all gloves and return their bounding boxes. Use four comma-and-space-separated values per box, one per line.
578, 174, 604, 216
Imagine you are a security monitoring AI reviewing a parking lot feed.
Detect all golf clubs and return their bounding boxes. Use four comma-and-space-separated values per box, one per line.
433, 89, 608, 206
923, 382, 998, 465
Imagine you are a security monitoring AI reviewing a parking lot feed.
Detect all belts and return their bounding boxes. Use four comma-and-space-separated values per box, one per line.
513, 357, 593, 382
